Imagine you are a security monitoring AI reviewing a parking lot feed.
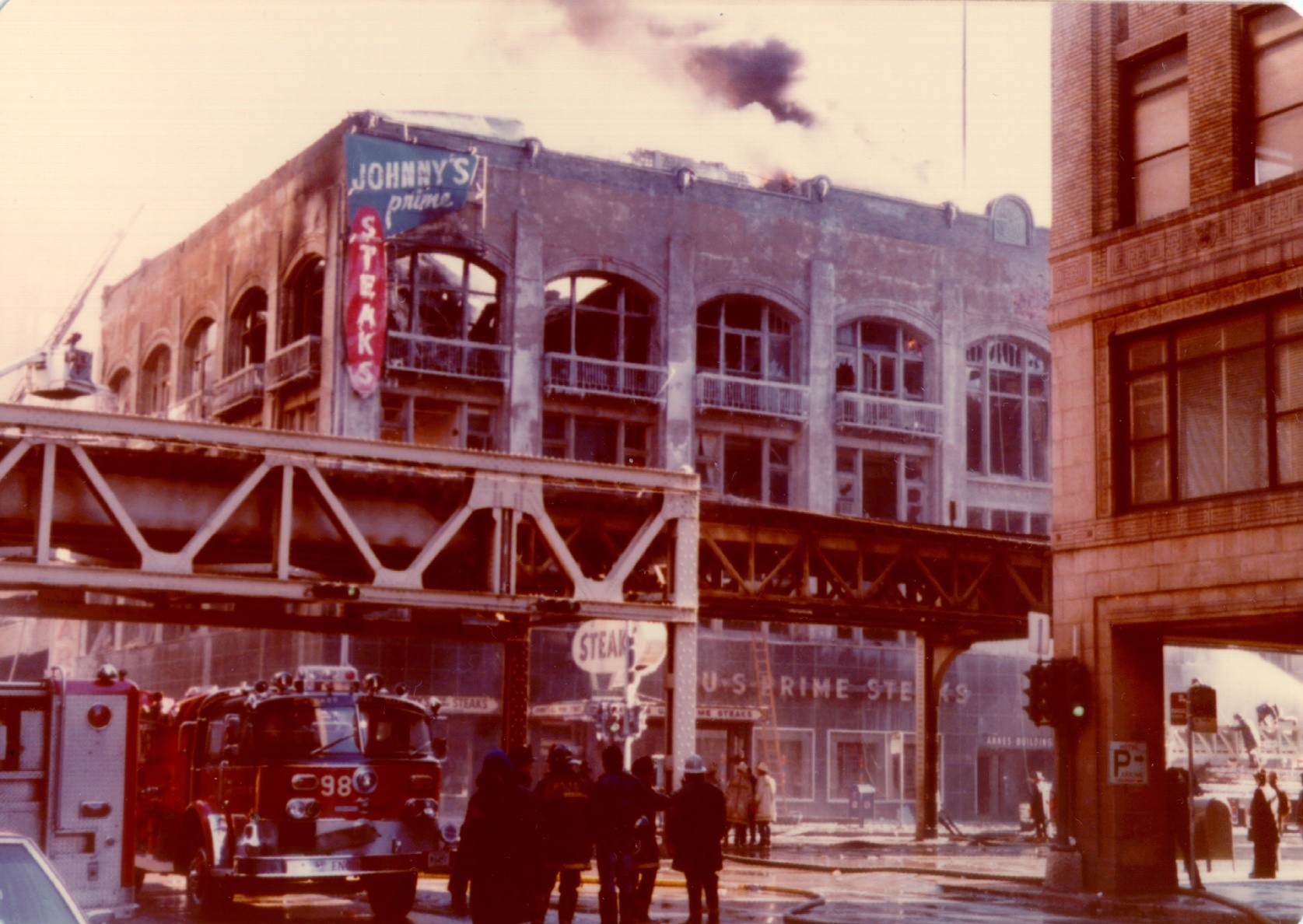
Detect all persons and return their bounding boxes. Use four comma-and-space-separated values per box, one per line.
705, 754, 779, 845
449, 744, 733, 924
1243, 767, 1290, 879
1027, 769, 1053, 842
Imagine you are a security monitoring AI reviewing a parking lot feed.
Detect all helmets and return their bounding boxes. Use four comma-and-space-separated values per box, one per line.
736, 761, 748, 773
545, 743, 574, 764
483, 748, 512, 780
680, 752, 707, 773
758, 761, 769, 773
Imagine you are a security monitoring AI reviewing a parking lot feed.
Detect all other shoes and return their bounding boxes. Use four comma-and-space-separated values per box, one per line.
723, 835, 771, 848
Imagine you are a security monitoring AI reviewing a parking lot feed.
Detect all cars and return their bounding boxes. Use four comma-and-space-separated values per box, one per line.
1189, 765, 1303, 831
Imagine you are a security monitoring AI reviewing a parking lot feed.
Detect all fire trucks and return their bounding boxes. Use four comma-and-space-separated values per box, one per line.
0, 663, 457, 924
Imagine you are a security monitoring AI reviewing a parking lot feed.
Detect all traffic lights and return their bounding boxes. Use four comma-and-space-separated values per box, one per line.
606, 702, 623, 742
1022, 664, 1045, 726
1060, 658, 1092, 737
